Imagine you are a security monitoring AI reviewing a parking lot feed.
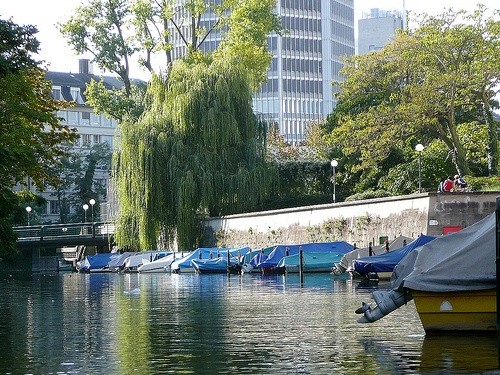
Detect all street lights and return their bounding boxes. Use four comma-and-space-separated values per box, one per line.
82, 203, 89, 223
89, 199, 97, 222
416, 144, 424, 193
26, 206, 31, 226
331, 159, 339, 203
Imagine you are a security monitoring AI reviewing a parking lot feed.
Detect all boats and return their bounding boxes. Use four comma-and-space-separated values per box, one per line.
79, 235, 438, 279
391, 212, 500, 333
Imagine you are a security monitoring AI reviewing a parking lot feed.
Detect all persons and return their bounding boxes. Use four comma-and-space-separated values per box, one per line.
381, 240, 389, 252
453, 175, 466, 192
403, 239, 407, 246
369, 242, 376, 256
436, 174, 454, 193
